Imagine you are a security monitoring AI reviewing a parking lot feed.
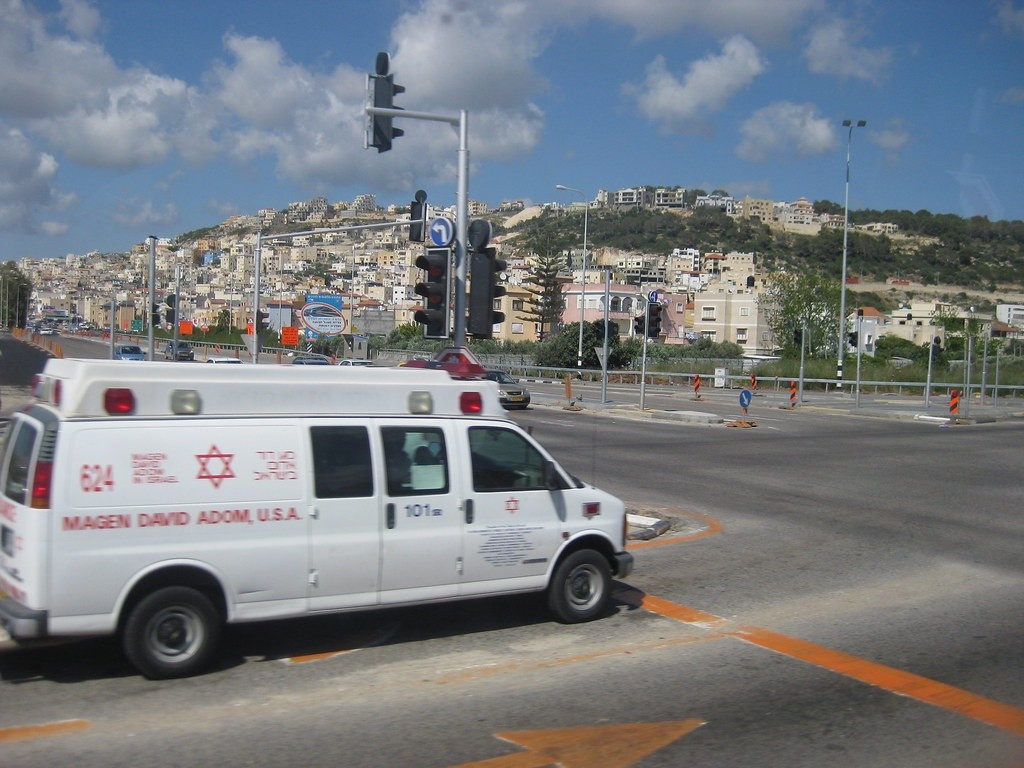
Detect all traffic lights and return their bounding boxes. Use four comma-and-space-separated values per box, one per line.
256, 309, 270, 334
634, 314, 645, 334
468, 248, 508, 339
933, 346, 944, 356
848, 331, 858, 347
793, 329, 802, 344
371, 72, 406, 153
415, 246, 452, 339
647, 302, 663, 339
153, 303, 160, 326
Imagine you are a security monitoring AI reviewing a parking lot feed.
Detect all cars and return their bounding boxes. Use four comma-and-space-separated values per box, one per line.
207, 356, 244, 365
26, 317, 115, 337
165, 341, 195, 362
481, 371, 530, 410
337, 359, 374, 368
293, 355, 331, 365
114, 345, 147, 362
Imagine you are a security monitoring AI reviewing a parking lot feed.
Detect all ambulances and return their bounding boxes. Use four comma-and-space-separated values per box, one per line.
0, 357, 638, 683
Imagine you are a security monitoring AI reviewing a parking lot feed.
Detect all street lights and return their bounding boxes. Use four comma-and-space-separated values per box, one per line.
555, 184, 588, 368
590, 263, 617, 403
836, 119, 867, 392
